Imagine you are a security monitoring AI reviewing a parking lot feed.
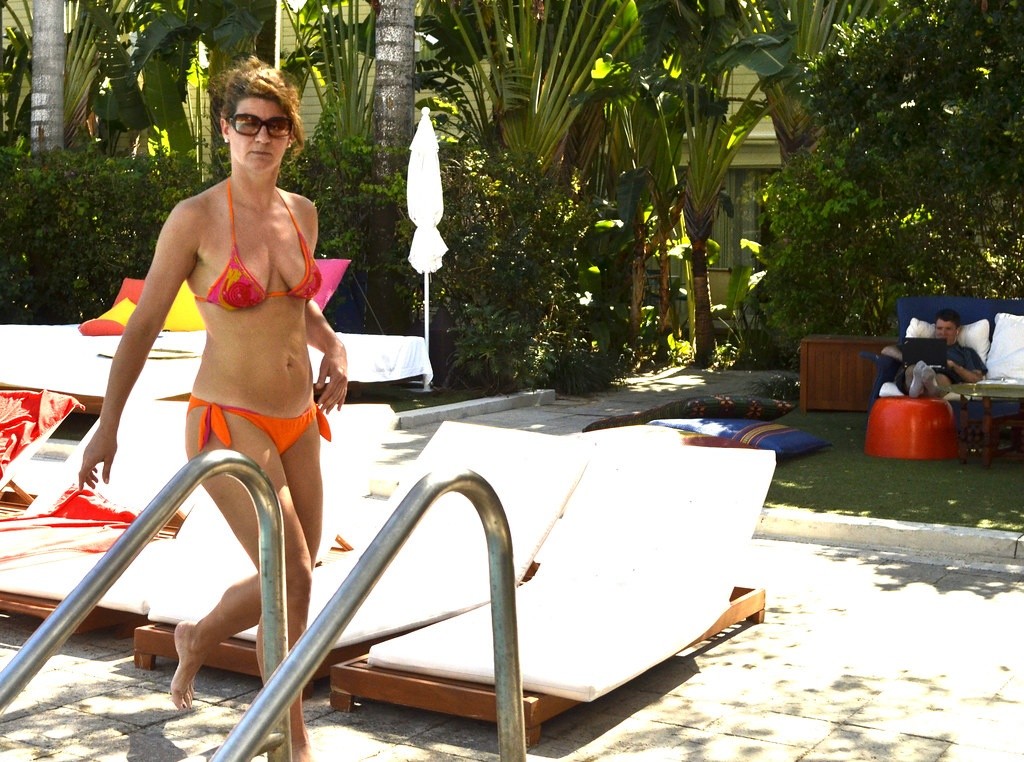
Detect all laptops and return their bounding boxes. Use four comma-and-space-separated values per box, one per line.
902, 337, 948, 373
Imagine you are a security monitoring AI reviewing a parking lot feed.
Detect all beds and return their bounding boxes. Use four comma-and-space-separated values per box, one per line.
0, 323, 434, 415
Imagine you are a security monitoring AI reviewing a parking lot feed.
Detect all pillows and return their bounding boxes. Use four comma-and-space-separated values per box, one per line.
313, 259, 352, 312
645, 417, 835, 456
160, 279, 208, 331
904, 317, 990, 368
78, 296, 138, 336
112, 277, 145, 306
984, 313, 1024, 381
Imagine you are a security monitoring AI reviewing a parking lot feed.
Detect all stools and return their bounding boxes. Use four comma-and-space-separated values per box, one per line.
864, 396, 960, 460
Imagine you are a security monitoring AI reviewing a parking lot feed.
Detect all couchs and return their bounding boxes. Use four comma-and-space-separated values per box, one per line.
860, 296, 1024, 426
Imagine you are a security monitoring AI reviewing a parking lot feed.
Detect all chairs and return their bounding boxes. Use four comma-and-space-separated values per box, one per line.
0, 389, 777, 751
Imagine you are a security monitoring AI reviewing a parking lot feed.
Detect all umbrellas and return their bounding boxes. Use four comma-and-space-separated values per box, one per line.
407, 108, 448, 389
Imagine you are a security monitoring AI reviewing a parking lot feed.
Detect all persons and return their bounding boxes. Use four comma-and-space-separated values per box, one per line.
881, 308, 988, 398
78, 57, 348, 762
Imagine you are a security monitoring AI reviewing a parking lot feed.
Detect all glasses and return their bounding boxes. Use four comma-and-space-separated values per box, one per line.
226, 114, 294, 137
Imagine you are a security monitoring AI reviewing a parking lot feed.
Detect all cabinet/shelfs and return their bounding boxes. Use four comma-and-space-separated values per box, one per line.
800, 333, 900, 416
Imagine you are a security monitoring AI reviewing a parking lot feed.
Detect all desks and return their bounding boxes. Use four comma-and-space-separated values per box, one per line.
951, 384, 1024, 467
